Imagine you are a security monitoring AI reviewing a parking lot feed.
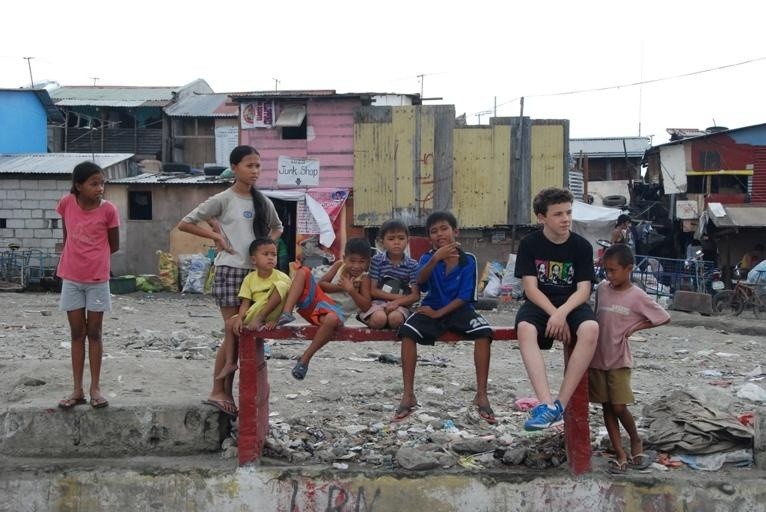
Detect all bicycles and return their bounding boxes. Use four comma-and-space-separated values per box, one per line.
712, 263, 766, 320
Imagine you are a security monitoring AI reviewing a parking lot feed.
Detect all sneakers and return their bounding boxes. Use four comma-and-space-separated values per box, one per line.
525, 399, 565, 431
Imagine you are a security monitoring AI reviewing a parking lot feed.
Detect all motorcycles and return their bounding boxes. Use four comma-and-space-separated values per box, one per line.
684, 250, 725, 298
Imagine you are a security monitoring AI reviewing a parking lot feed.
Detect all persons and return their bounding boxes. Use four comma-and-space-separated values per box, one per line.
588, 243, 671, 474
273, 237, 290, 276
353, 217, 421, 330
56, 160, 121, 409
686, 238, 705, 293
513, 185, 601, 431
740, 242, 766, 271
214, 237, 295, 381
393, 210, 498, 425
609, 214, 631, 245
178, 144, 284, 417
276, 236, 372, 380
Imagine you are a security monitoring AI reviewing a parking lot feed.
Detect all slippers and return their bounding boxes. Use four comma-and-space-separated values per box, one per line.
292, 358, 308, 380
58, 397, 86, 409
476, 407, 496, 424
202, 399, 239, 418
90, 398, 109, 408
276, 311, 295, 325
609, 454, 629, 474
393, 401, 420, 419
629, 449, 657, 470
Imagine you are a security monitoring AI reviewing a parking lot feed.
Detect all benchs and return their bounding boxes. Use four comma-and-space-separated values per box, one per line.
239, 325, 591, 473
